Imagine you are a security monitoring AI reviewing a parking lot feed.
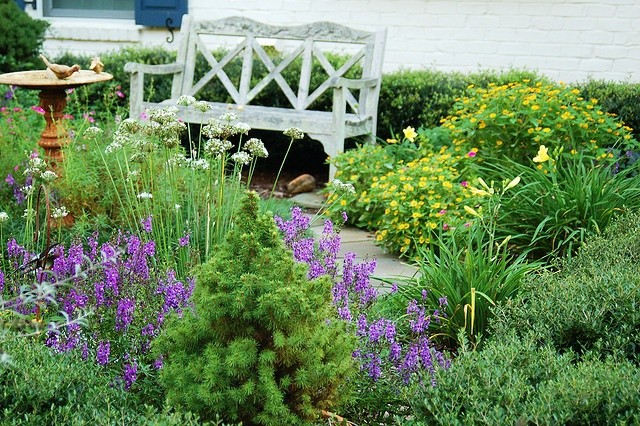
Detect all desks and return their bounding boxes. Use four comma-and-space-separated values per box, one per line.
0, 70, 116, 229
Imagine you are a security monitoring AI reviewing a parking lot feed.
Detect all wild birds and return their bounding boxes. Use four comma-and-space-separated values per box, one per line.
39, 53, 81, 79
89, 57, 105, 74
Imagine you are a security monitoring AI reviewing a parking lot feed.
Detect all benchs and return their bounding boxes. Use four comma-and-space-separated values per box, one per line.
123, 13, 386, 200
